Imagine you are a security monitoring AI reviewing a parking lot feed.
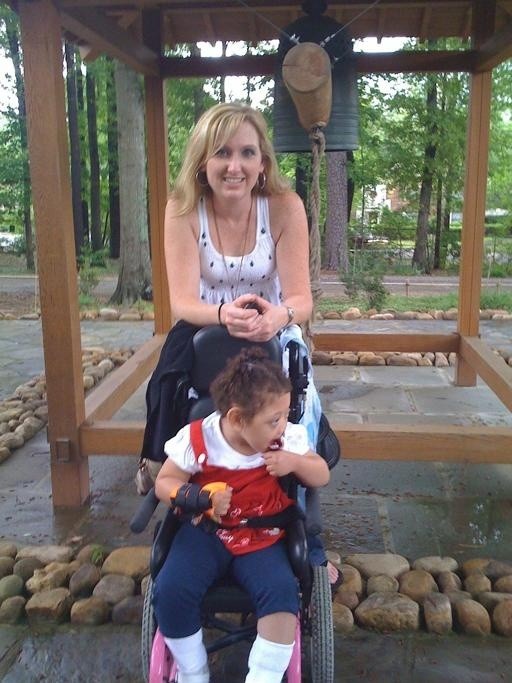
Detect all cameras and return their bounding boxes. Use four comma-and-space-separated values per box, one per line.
243, 302, 263, 315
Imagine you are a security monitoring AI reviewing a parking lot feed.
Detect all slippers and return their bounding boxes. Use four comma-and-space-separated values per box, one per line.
320, 560, 343, 589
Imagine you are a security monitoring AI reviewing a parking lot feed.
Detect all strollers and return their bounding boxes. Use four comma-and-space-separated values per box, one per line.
128, 322, 333, 682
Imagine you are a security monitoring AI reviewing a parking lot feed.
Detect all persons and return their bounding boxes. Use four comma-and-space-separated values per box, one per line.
148, 346, 331, 683
134, 101, 344, 591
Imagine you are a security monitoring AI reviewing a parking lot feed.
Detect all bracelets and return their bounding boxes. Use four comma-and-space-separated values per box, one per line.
282, 305, 295, 328
217, 303, 224, 327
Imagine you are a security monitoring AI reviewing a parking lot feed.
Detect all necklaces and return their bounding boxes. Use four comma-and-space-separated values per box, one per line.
209, 198, 253, 303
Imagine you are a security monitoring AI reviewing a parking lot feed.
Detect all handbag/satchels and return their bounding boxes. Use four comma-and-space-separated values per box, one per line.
135, 457, 162, 495
